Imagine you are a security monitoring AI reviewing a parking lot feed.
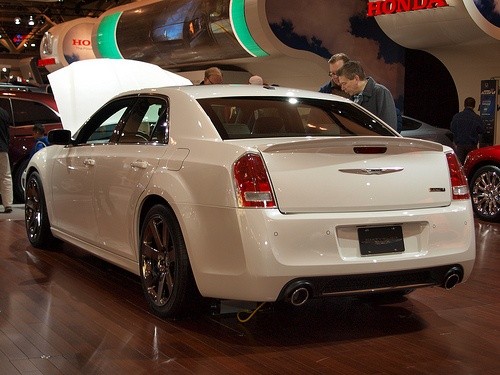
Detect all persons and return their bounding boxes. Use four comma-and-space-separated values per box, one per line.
336, 60, 397, 131
318, 53, 352, 99
0, 107, 13, 213
32, 123, 50, 153
395, 108, 402, 133
450, 97, 486, 166
199, 67, 225, 86
249, 76, 264, 86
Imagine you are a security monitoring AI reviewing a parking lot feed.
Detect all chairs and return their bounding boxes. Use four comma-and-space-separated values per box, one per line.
252, 117, 283, 133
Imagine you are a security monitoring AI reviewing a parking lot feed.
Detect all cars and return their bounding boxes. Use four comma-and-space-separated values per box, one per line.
463, 144, 500, 223
396, 115, 455, 150
21, 57, 477, 315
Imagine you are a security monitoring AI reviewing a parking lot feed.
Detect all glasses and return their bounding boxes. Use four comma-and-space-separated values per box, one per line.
328, 70, 339, 76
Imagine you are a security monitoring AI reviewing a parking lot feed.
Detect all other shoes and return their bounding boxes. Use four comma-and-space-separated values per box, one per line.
5, 206, 13, 212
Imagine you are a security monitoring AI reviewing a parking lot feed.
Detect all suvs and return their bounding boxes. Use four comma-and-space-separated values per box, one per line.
0, 83, 70, 185
